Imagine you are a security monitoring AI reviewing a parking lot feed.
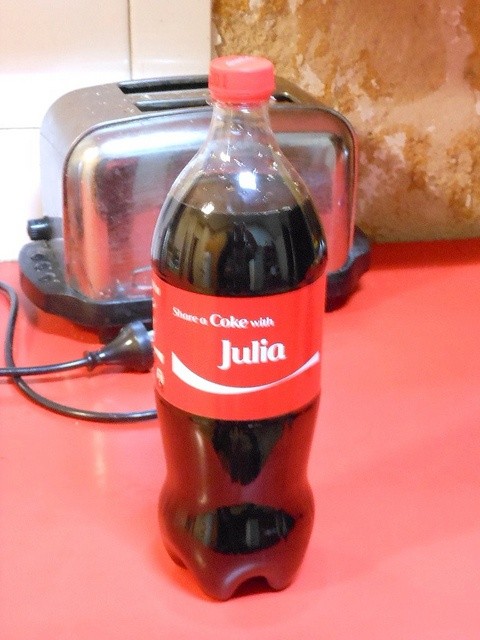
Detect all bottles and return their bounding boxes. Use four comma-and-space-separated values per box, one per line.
150, 54, 328, 601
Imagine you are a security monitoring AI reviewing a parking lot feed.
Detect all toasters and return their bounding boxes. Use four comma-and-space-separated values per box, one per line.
18, 74, 372, 328
